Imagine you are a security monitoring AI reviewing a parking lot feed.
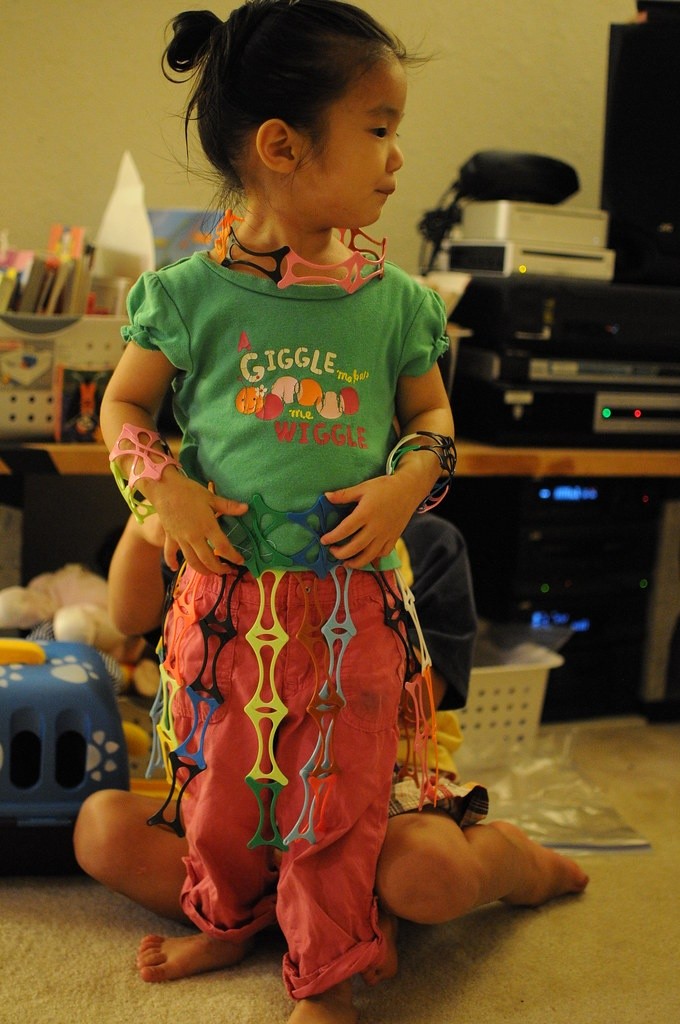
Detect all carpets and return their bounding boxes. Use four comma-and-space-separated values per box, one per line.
444, 745, 654, 848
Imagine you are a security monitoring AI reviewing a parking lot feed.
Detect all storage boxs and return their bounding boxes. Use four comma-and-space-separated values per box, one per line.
440, 617, 567, 774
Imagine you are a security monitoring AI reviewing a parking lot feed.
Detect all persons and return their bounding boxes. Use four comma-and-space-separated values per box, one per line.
73, 0, 589, 1024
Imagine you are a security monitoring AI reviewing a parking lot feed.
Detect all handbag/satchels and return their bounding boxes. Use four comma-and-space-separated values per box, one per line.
417, 149, 580, 276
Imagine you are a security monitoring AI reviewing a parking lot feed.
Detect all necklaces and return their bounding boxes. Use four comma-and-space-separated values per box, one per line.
214, 209, 386, 294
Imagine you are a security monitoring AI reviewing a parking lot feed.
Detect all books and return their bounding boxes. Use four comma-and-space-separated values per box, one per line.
0, 221, 96, 317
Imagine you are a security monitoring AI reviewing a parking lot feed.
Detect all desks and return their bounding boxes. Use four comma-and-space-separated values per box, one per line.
0, 435, 680, 726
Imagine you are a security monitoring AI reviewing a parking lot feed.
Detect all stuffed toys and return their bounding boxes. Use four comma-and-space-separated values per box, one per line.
0, 565, 127, 697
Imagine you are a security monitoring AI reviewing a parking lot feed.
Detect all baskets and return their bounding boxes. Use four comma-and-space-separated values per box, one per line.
446, 639, 566, 756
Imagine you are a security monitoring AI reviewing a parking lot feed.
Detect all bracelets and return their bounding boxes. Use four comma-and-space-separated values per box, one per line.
108, 422, 188, 526
386, 431, 456, 513
